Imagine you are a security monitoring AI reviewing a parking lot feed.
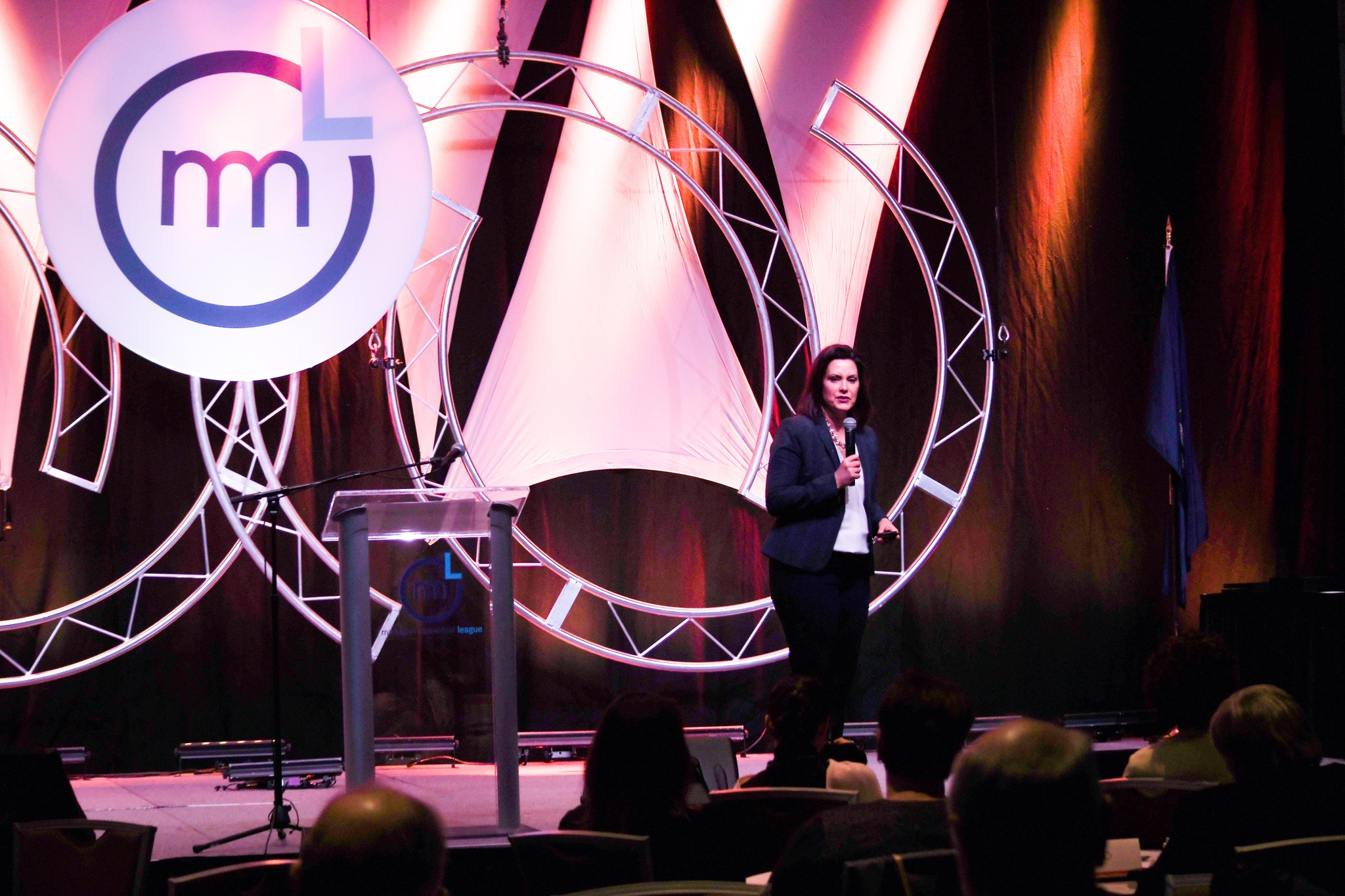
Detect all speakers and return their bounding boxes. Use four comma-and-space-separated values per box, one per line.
684, 738, 736, 790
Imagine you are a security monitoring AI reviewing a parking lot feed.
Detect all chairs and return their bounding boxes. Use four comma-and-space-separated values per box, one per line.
15, 761, 1345, 896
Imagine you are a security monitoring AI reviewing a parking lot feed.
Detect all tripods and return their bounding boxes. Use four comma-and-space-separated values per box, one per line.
192, 457, 434, 853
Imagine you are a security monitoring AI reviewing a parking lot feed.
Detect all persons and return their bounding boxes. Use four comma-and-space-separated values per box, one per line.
759, 344, 902, 739
1138, 685, 1344, 896
945, 719, 1109, 896
290, 788, 444, 896
556, 693, 703, 849
876, 667, 975, 801
1123, 637, 1235, 782
732, 680, 884, 803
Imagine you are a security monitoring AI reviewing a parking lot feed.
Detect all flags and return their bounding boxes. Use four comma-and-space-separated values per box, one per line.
1147, 255, 1210, 612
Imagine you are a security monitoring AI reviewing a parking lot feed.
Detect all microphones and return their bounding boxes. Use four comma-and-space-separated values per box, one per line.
843, 418, 857, 486
440, 443, 465, 469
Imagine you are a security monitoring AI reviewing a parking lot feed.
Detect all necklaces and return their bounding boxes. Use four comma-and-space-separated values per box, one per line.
826, 420, 847, 449
